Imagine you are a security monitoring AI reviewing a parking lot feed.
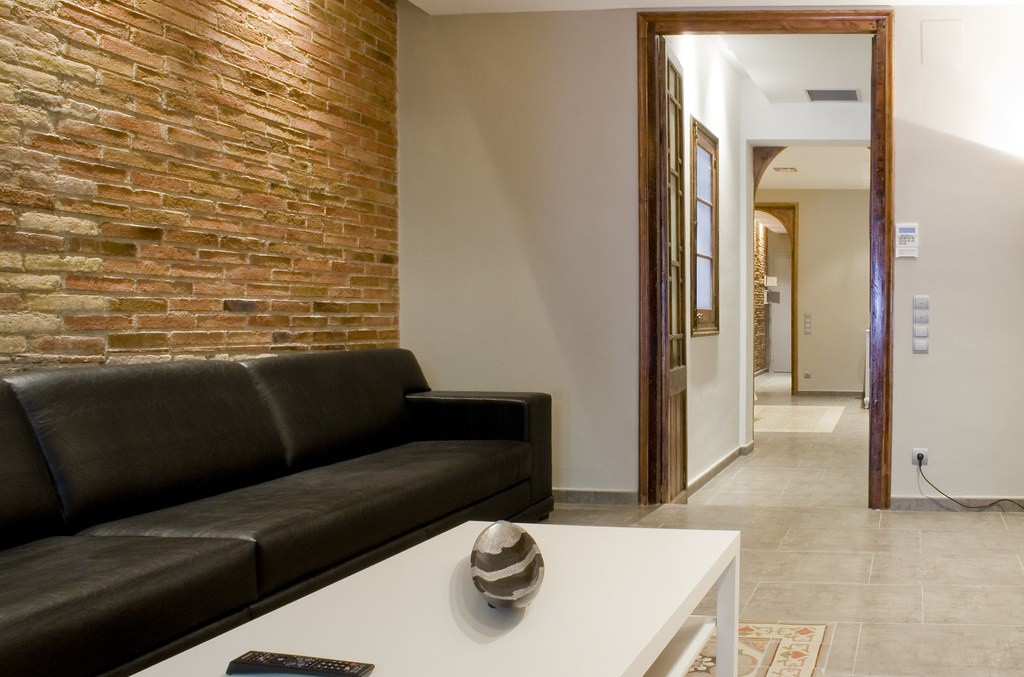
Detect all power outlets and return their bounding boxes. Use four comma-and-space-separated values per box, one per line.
912, 448, 928, 465
804, 371, 811, 378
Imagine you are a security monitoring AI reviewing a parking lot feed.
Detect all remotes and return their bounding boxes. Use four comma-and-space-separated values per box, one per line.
225, 651, 376, 677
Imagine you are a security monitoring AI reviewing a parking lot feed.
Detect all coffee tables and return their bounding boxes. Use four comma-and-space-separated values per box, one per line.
127, 521, 741, 677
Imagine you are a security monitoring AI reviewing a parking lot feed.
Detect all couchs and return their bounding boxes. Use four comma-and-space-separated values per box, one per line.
0, 350, 556, 677
